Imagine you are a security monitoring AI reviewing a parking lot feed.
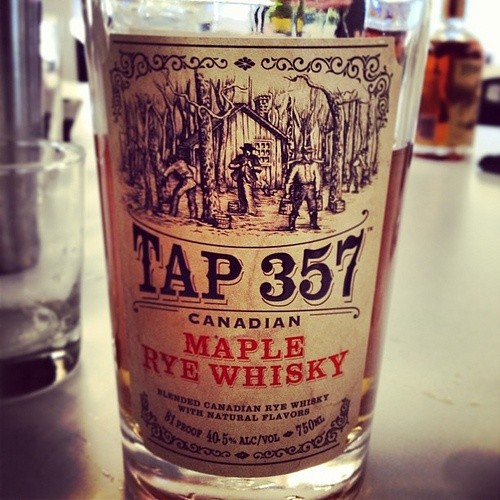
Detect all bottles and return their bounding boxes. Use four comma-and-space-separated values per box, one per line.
415, 0, 484, 162
79, 0, 430, 500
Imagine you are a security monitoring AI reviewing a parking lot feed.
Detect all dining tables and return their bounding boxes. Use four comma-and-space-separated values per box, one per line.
1, 123, 500, 500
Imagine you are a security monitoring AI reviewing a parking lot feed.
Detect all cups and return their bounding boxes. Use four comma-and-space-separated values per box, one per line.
1, 139, 89, 405
